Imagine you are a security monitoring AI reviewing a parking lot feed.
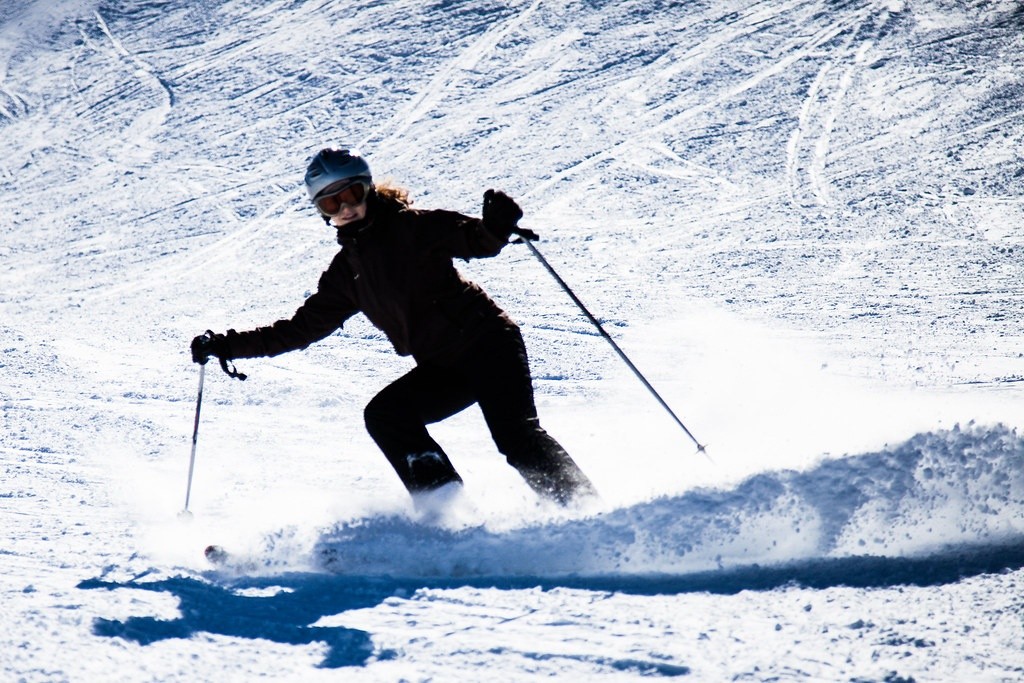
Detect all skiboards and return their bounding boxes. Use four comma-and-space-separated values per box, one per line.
201, 544, 340, 573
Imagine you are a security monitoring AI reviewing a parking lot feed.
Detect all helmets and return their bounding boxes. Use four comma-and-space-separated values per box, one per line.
305, 148, 372, 204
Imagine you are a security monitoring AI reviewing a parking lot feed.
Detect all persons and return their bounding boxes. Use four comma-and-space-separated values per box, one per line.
189, 148, 606, 516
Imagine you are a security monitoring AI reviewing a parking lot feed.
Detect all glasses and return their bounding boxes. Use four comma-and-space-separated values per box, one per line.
314, 178, 370, 216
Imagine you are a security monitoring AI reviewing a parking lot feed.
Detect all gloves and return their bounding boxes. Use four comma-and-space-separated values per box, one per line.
482, 190, 523, 239
190, 335, 212, 365
